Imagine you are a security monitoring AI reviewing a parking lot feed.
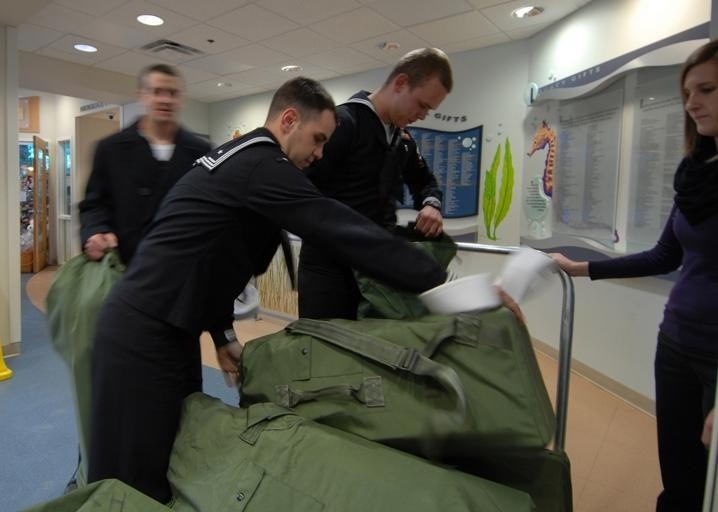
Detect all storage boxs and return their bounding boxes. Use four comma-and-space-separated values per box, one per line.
20, 248, 33, 273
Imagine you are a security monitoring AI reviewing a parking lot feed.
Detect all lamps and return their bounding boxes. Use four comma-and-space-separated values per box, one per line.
146, 44, 198, 65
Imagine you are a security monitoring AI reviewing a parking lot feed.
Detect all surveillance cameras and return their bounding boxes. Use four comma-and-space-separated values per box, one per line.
109, 114, 115, 119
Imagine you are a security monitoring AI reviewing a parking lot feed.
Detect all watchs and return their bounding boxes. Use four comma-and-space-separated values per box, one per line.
422, 199, 442, 213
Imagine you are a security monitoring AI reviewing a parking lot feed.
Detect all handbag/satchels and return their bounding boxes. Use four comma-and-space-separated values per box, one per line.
348, 227, 462, 319
236, 308, 555, 450
50, 251, 124, 380
47, 391, 572, 512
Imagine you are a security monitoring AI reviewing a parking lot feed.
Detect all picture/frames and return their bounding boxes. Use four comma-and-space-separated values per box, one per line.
396, 125, 483, 218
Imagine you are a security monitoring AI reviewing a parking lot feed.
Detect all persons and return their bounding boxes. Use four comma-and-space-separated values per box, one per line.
293, 47, 453, 320
87, 75, 460, 506
76, 63, 216, 396
542, 39, 717, 511
